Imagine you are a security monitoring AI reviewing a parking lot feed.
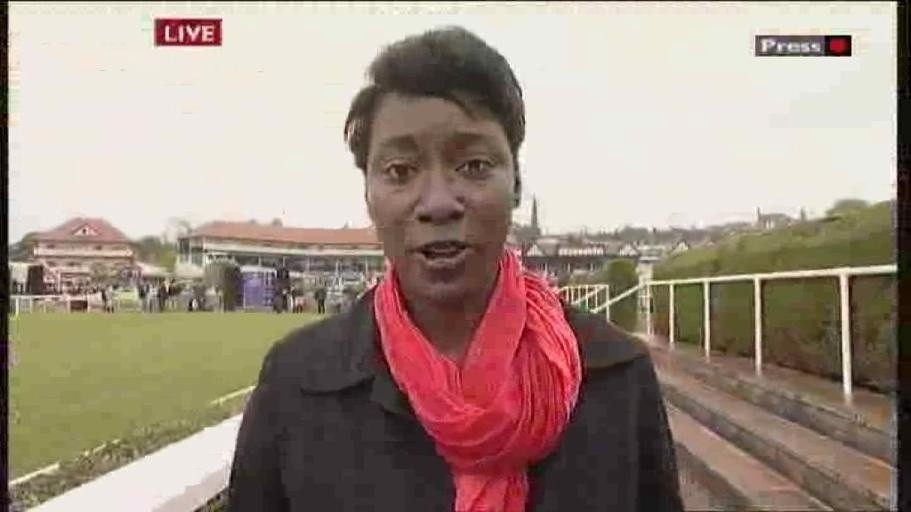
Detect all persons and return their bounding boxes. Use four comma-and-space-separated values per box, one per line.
49, 271, 225, 316
272, 266, 305, 315
314, 284, 328, 315
225, 18, 688, 512
